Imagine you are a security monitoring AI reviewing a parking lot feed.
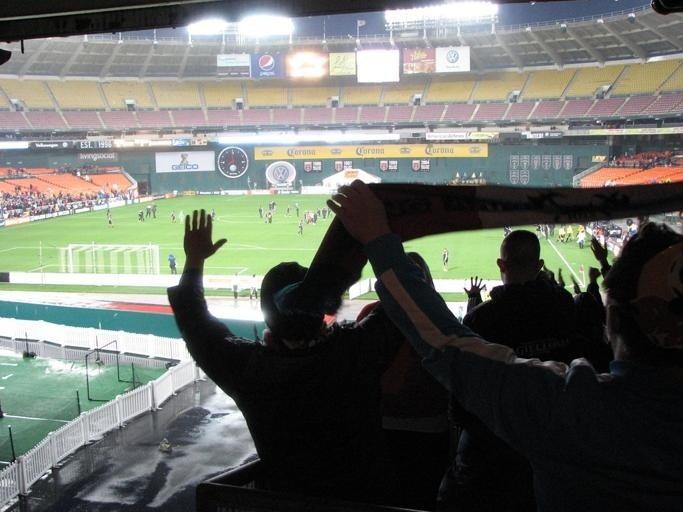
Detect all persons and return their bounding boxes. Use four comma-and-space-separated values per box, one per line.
167, 179, 683, 512
0, 161, 158, 228
607, 148, 674, 168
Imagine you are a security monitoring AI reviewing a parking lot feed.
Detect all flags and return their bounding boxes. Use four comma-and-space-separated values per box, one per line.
216, 43, 471, 83
